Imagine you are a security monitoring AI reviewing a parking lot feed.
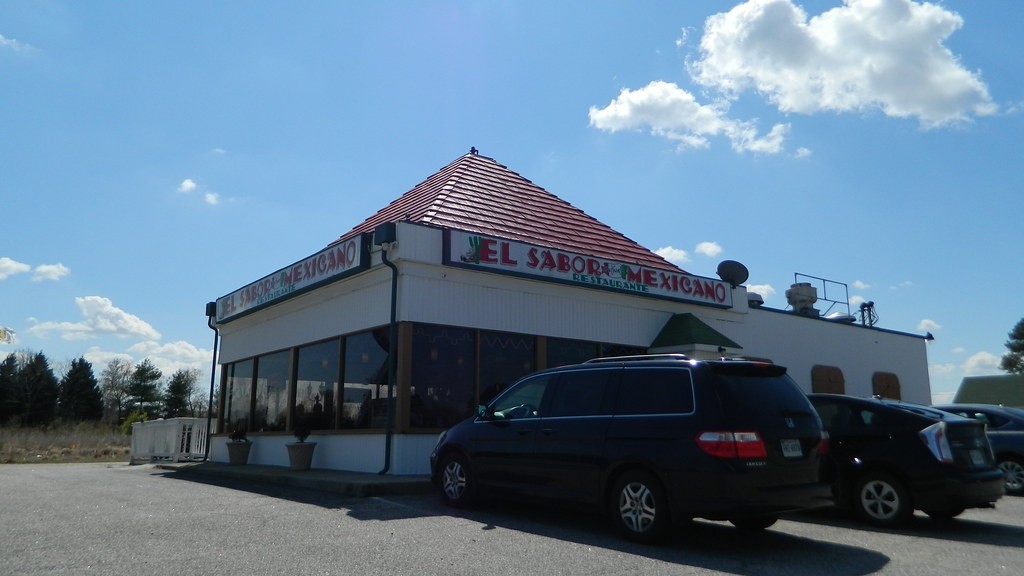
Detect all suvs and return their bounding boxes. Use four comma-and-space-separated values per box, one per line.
437, 352, 839, 543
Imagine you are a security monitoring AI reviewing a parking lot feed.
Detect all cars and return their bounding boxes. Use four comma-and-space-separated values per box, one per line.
798, 391, 1006, 525
928, 404, 1024, 494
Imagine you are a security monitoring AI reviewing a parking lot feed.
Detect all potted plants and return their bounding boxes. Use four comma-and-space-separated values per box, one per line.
285, 415, 317, 471
225, 429, 253, 465
